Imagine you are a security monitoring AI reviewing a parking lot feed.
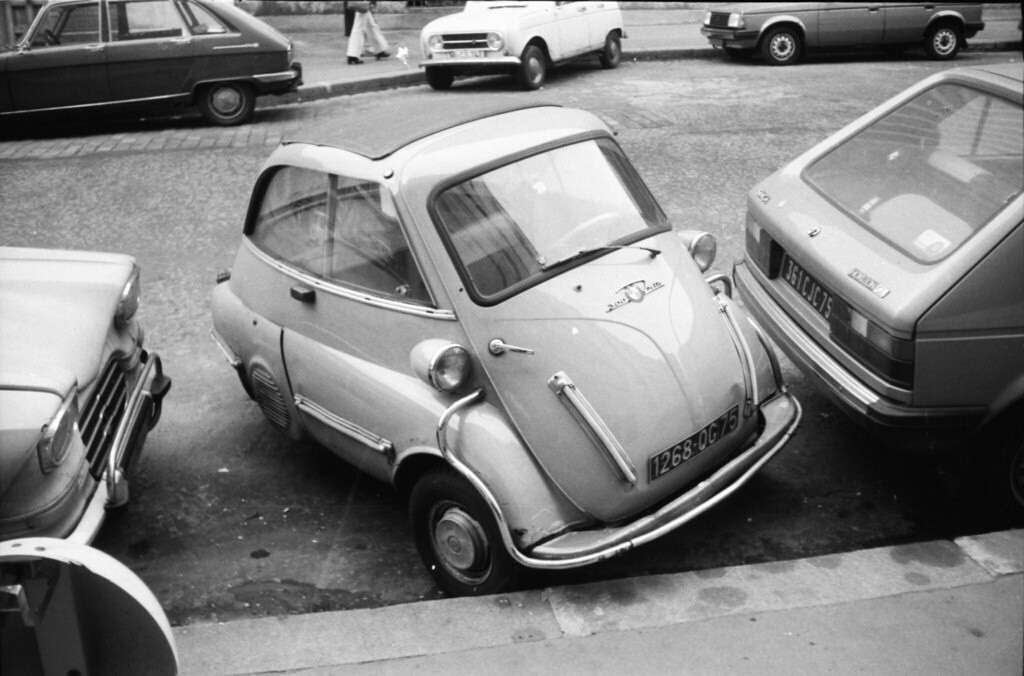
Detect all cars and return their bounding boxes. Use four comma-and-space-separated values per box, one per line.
0, 238, 173, 565
418, 0, 627, 91
732, 59, 1023, 478
0, 0, 306, 141
207, 92, 804, 603
703, 2, 987, 67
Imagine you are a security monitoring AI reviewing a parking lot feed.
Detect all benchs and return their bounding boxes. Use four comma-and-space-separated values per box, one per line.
927, 146, 1018, 210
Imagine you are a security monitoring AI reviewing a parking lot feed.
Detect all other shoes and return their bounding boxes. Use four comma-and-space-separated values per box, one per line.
347, 56, 364, 64
375, 51, 390, 60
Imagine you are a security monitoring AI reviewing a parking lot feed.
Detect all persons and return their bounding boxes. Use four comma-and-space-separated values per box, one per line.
346, 0, 391, 64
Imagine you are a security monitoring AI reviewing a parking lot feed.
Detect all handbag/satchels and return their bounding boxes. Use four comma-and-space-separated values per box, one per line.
346, 0, 370, 11
